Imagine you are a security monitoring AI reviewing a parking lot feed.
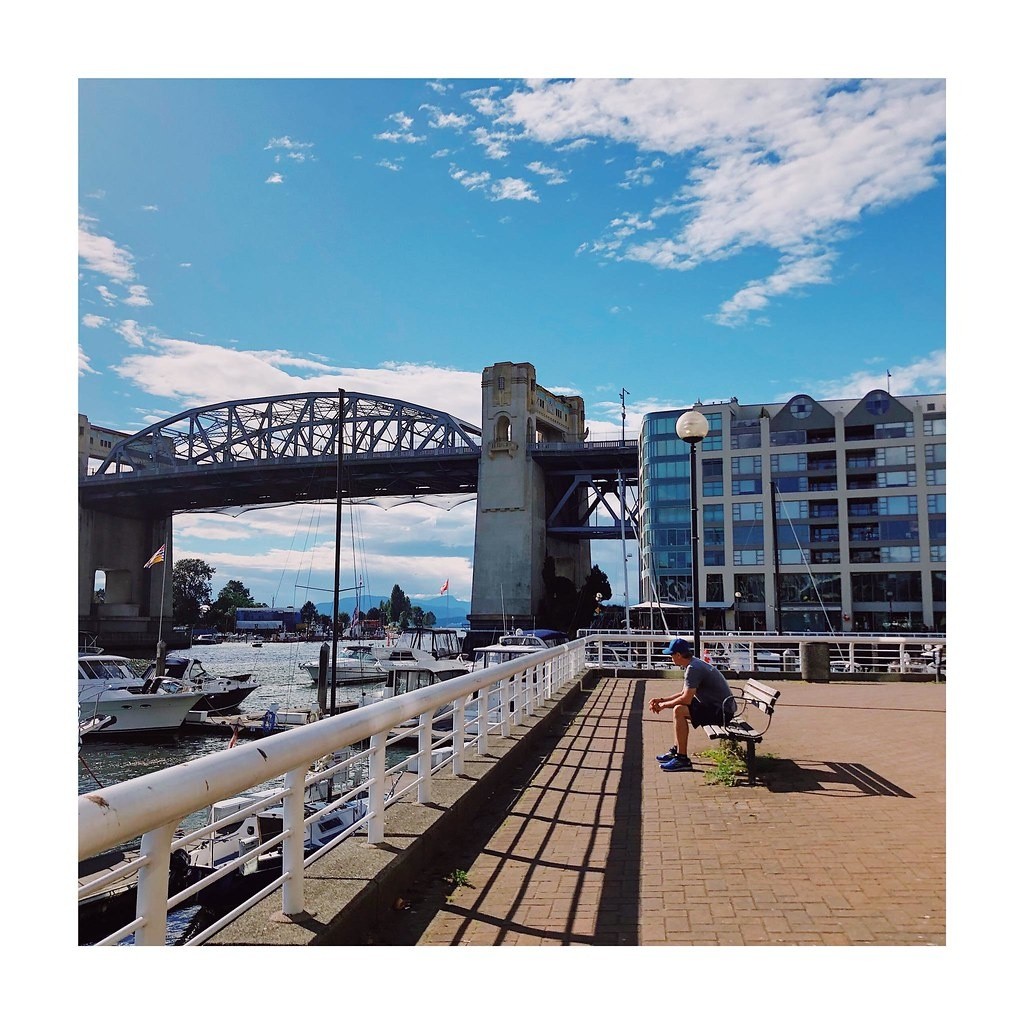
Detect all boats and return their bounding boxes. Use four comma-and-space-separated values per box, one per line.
79, 655, 207, 734
252, 642, 261, 648
78, 844, 162, 909
140, 656, 264, 715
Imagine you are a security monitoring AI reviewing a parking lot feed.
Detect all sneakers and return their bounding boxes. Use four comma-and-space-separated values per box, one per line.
656, 746, 677, 762
659, 754, 693, 771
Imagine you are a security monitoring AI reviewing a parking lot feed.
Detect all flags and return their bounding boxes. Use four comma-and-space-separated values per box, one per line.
441, 579, 448, 595
144, 543, 165, 568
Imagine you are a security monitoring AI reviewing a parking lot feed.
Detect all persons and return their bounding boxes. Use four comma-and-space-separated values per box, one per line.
649, 638, 737, 771
855, 615, 870, 632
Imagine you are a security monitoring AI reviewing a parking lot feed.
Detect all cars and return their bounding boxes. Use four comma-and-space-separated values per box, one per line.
197, 634, 216, 642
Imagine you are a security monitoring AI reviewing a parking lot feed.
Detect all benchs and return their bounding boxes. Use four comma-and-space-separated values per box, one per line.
702, 677, 781, 786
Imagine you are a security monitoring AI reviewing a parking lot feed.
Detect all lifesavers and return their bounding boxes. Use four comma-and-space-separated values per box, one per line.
262, 710, 275, 735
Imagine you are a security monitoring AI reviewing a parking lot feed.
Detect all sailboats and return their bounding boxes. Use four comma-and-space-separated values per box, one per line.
208, 627, 572, 736
168, 387, 383, 880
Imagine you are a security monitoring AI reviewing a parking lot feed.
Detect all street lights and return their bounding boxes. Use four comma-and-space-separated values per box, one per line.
618, 387, 631, 441
676, 411, 708, 659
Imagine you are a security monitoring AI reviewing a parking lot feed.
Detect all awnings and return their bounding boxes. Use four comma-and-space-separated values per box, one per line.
666, 602, 734, 610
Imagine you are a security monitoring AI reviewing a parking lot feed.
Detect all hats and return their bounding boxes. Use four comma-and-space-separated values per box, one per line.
662, 638, 690, 655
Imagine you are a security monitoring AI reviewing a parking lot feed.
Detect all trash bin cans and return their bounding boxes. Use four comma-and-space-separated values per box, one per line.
799, 640, 831, 684
